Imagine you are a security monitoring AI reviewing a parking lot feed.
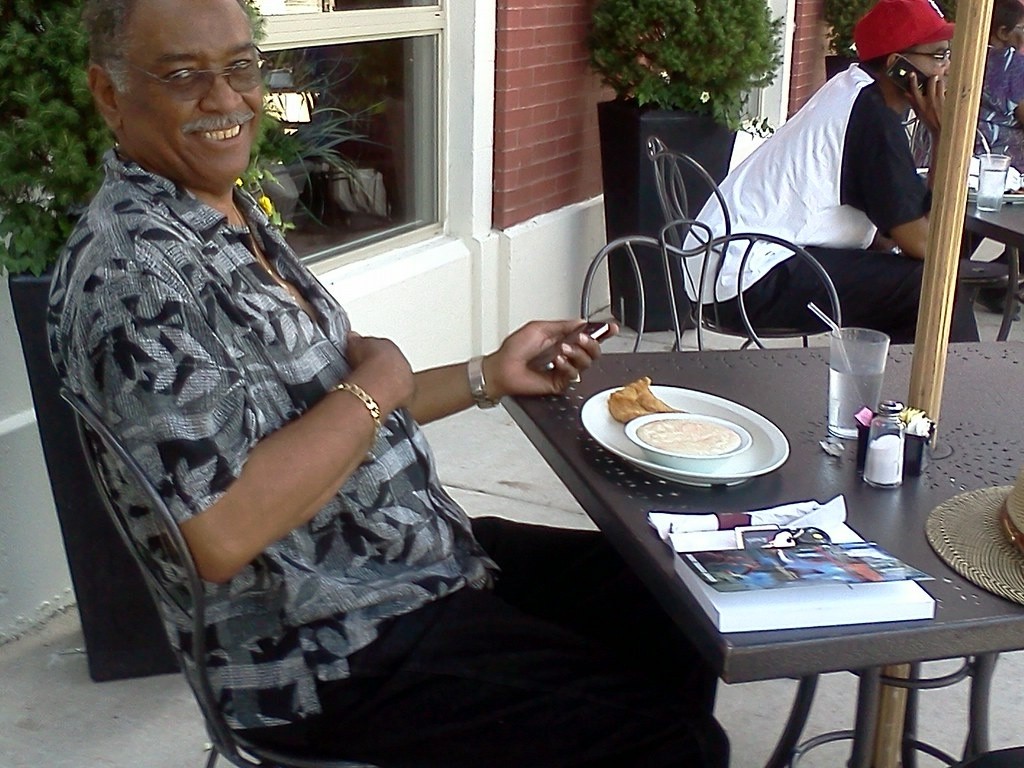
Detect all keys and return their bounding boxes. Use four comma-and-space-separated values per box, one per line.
764, 526, 832, 548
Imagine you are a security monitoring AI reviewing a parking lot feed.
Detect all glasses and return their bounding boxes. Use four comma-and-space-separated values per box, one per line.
119, 47, 269, 102
899, 48, 952, 68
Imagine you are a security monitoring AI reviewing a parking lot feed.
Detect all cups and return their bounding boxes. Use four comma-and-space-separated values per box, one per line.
825, 326, 890, 440
976, 154, 1011, 212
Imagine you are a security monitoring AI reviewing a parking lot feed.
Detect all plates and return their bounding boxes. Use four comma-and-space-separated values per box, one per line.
967, 191, 1024, 206
580, 384, 791, 487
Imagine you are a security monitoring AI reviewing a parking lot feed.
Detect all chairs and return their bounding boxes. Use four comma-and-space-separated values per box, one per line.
579, 136, 841, 351
57, 385, 382, 768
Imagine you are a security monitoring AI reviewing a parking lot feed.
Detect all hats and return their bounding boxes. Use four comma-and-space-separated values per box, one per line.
925, 469, 1023, 608
853, 0, 956, 62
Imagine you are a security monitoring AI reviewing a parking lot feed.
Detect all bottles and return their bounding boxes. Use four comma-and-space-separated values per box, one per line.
863, 400, 907, 489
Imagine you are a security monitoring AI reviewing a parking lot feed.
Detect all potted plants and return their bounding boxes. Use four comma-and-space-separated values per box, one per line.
588, 0, 785, 332
0, 0, 270, 682
825, 0, 874, 82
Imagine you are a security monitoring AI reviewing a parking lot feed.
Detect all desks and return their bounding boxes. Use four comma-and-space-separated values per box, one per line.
497, 339, 1024, 768
920, 168, 1024, 341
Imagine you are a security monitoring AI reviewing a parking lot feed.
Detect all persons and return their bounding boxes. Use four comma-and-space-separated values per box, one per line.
44, 0, 736, 767
681, 0, 981, 345
912, 0, 1024, 178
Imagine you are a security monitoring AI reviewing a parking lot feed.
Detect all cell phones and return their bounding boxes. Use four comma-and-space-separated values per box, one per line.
527, 322, 609, 371
884, 56, 948, 97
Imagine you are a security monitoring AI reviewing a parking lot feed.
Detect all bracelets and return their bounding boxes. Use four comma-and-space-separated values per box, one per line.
467, 355, 501, 410
328, 383, 382, 448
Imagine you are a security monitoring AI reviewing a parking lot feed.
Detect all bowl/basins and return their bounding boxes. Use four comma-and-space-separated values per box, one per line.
623, 412, 753, 474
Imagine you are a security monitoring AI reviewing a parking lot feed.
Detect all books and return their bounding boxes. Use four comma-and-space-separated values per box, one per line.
673, 542, 939, 633
